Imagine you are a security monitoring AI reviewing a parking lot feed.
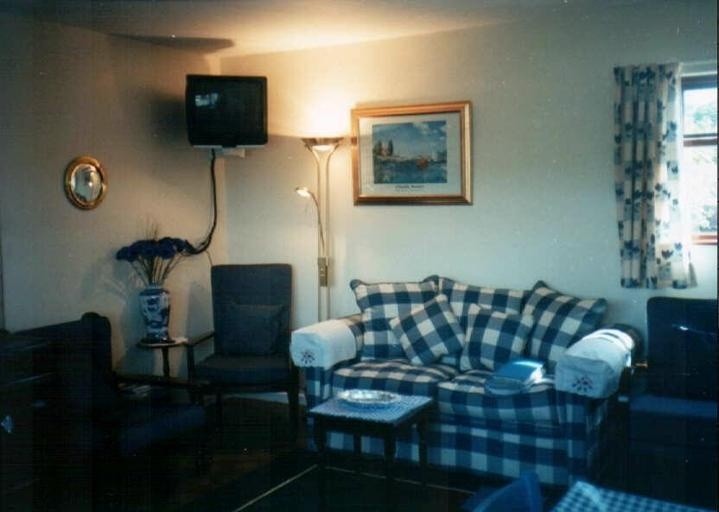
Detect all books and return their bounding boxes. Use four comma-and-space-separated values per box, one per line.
492, 358, 546, 385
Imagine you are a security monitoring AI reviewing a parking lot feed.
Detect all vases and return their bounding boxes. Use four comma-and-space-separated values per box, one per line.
136, 282, 174, 342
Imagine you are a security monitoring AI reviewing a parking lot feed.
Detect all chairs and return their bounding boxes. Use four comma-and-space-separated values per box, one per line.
0, 311, 209, 512
184, 261, 304, 451
606, 295, 719, 506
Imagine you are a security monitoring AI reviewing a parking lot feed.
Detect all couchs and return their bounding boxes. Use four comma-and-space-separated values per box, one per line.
287, 314, 642, 492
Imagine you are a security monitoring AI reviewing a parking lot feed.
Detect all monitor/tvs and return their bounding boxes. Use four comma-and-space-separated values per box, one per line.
185, 74, 267, 145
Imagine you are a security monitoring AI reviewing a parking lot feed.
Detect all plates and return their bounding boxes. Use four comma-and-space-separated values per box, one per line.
336, 388, 401, 408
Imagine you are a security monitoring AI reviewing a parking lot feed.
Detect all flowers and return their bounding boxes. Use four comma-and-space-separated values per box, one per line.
112, 235, 197, 287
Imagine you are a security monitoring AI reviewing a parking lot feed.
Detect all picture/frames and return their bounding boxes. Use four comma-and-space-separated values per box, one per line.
348, 99, 474, 206
64, 155, 111, 211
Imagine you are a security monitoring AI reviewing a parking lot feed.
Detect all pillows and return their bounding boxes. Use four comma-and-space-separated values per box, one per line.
348, 273, 609, 375
222, 299, 284, 357
662, 319, 718, 400
